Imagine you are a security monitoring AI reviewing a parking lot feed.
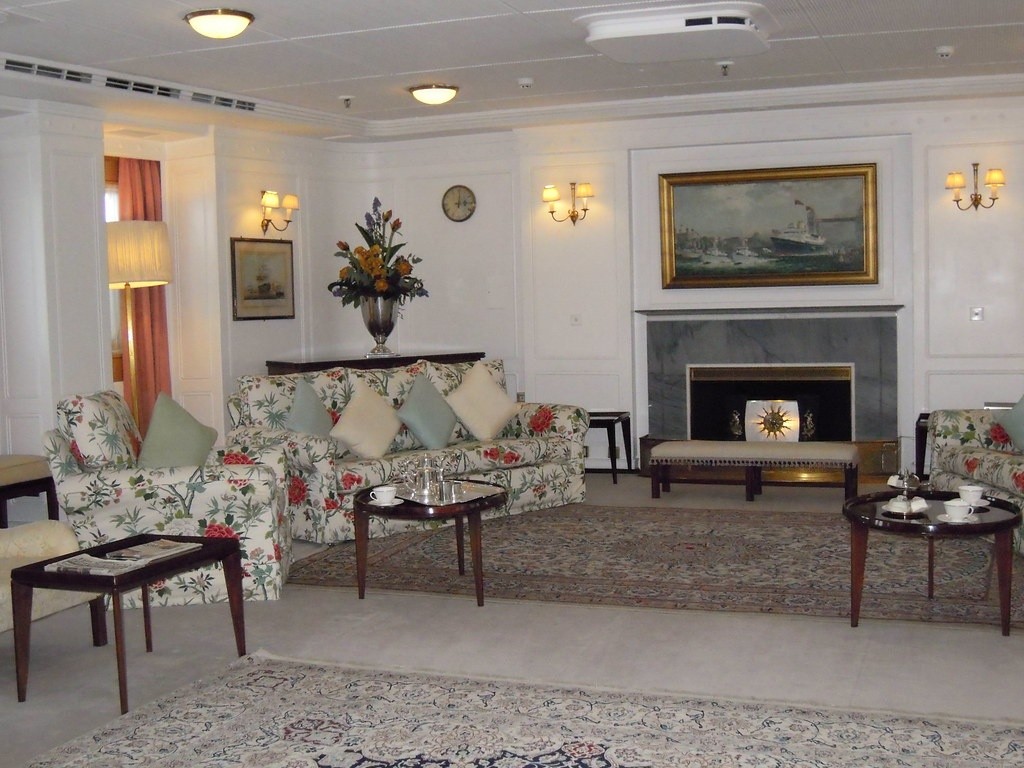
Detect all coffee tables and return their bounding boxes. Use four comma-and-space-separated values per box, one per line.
355, 478, 509, 607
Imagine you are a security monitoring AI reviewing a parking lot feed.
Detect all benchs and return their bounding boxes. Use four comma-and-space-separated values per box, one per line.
648, 438, 861, 505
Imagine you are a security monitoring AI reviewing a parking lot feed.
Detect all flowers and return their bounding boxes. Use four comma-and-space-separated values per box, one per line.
327, 197, 430, 309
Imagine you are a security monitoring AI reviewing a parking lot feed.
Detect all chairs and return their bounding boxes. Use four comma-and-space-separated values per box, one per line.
0, 451, 60, 528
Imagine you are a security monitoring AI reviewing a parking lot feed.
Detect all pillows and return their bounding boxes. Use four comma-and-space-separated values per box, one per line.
287, 360, 523, 460
138, 391, 217, 468
996, 395, 1024, 452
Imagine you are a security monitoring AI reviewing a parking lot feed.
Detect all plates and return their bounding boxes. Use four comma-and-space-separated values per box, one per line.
951, 497, 990, 510
368, 498, 404, 507
882, 504, 932, 515
937, 514, 977, 524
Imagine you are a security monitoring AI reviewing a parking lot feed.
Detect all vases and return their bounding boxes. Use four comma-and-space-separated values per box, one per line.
357, 295, 400, 358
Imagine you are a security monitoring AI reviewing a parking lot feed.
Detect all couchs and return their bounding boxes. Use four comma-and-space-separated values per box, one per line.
227, 359, 591, 548
0, 520, 105, 632
927, 408, 1024, 553
42, 389, 294, 611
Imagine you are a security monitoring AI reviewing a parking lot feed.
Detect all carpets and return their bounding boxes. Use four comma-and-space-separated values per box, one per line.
19, 648, 1024, 768
284, 502, 1023, 630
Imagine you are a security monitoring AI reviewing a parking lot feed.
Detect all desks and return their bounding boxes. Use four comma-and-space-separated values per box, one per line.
12, 534, 248, 714
915, 414, 931, 481
589, 410, 632, 484
842, 490, 1022, 637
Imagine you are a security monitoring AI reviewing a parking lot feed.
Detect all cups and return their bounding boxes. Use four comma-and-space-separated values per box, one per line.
370, 486, 397, 504
889, 496, 928, 512
887, 475, 904, 487
959, 486, 982, 503
945, 498, 974, 520
438, 481, 461, 501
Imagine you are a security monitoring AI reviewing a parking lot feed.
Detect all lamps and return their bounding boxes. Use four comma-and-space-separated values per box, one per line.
944, 162, 1004, 210
542, 181, 595, 226
184, 9, 256, 38
409, 84, 458, 106
260, 189, 299, 236
106, 218, 173, 428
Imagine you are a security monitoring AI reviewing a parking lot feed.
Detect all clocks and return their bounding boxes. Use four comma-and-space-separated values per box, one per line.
441, 185, 476, 222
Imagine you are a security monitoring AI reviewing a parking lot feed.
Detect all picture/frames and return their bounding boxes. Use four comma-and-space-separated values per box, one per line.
659, 163, 878, 291
230, 236, 296, 321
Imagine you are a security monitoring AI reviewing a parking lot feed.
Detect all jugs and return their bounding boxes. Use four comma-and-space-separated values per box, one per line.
403, 458, 445, 496
403, 453, 444, 471
